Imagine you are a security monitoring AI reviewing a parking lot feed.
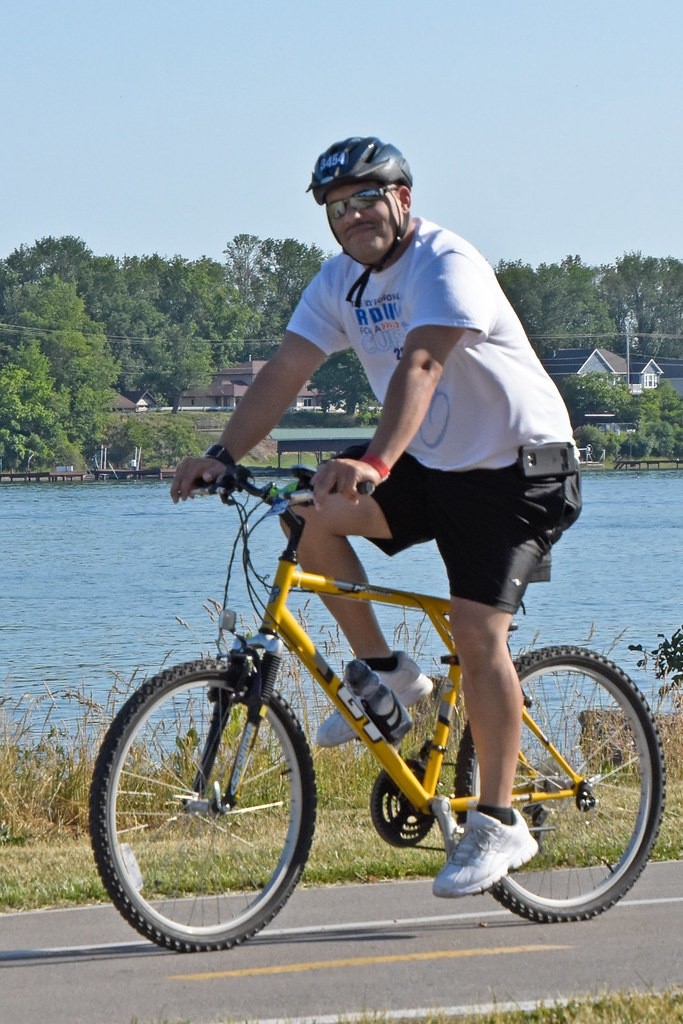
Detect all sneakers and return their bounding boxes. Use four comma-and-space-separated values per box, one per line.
317, 652, 433, 748
431, 805, 538, 897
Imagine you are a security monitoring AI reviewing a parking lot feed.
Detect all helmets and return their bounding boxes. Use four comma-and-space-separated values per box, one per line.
311, 137, 413, 206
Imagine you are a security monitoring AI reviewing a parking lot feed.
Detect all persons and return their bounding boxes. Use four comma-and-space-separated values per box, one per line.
172, 136, 584, 899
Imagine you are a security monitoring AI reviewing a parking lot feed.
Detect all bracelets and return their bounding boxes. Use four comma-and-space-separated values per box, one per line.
360, 450, 390, 485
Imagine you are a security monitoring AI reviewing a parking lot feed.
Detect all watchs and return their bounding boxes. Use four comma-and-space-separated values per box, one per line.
202, 443, 237, 473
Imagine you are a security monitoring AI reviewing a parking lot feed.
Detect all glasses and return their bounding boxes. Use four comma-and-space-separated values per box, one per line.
325, 184, 401, 218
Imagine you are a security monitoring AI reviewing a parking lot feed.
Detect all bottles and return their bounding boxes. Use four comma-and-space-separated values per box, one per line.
345, 660, 413, 741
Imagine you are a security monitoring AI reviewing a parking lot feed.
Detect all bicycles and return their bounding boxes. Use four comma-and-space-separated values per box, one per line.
88, 464, 667, 954
